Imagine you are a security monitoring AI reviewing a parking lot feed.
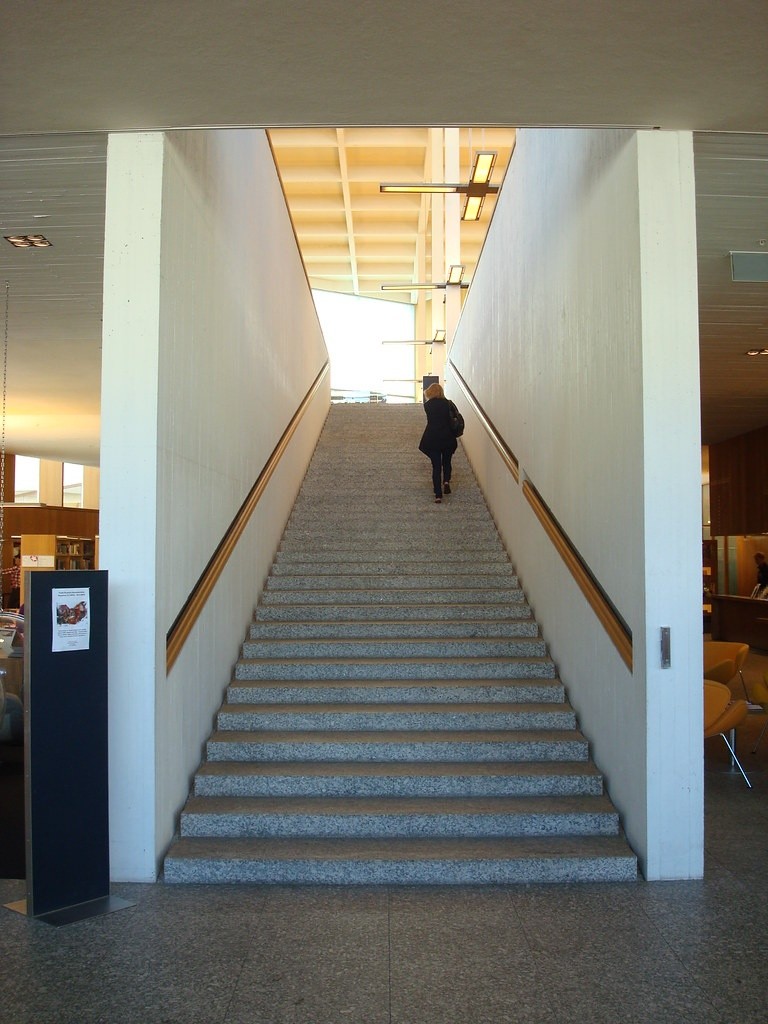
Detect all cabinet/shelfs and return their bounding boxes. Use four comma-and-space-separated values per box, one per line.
21, 534, 94, 607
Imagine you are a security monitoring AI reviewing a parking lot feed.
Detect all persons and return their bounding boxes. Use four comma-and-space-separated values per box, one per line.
0, 555, 20, 609
418, 383, 458, 503
754, 554, 767, 589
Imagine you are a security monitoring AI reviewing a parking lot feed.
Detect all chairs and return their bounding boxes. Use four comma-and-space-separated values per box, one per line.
703, 641, 753, 790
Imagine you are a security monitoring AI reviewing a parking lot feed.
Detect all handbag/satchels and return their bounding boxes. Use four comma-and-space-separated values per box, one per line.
448, 400, 465, 437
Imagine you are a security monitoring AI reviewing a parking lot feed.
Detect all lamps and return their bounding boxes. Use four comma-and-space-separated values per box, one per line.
433, 329, 446, 342
461, 151, 498, 221
380, 282, 446, 290
430, 346, 432, 355
447, 265, 466, 285
382, 340, 433, 345
460, 281, 470, 289
378, 128, 501, 212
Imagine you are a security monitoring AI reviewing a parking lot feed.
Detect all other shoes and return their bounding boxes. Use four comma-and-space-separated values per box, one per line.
444, 484, 451, 494
435, 500, 441, 503
